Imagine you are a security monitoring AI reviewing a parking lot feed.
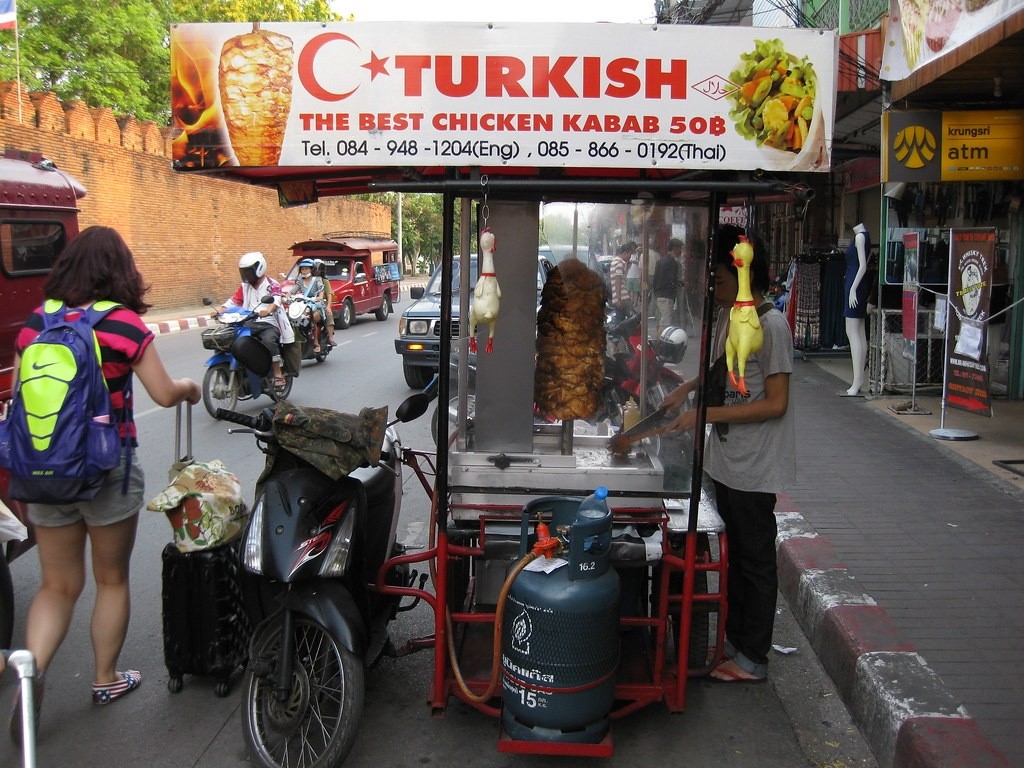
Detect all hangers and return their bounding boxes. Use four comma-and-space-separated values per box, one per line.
865, 246, 879, 267
793, 248, 832, 263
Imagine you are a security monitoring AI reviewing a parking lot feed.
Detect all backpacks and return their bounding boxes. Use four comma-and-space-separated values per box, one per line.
0, 298, 123, 503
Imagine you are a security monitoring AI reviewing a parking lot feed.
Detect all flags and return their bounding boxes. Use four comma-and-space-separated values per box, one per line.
0, 0, 17, 31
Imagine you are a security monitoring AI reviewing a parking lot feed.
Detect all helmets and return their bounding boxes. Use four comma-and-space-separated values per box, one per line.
314, 258, 326, 277
299, 258, 318, 276
239, 251, 267, 277
658, 326, 688, 357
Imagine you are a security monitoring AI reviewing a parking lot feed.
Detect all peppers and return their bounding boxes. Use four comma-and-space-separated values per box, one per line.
726, 38, 818, 149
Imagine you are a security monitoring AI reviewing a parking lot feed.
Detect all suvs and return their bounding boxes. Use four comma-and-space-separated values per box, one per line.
395, 253, 555, 389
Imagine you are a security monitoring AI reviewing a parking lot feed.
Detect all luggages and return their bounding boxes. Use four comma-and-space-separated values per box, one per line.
161, 396, 245, 698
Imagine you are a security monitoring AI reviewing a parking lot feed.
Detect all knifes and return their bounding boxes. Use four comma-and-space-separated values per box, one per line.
618, 408, 666, 437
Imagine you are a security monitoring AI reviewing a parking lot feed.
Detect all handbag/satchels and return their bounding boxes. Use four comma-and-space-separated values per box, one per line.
148, 456, 254, 552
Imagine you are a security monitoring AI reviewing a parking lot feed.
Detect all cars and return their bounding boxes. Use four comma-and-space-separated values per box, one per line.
538, 244, 611, 283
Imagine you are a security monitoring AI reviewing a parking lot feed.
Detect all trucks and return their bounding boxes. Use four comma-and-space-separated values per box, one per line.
281, 231, 404, 330
0, 146, 87, 415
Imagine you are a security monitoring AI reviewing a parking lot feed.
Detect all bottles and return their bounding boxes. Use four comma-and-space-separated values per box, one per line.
623, 396, 640, 446
93, 414, 117, 469
568, 487, 609, 553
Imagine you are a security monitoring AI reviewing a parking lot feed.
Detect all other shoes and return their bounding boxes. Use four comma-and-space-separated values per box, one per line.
313, 344, 321, 353
92, 669, 143, 705
10, 671, 45, 746
327, 340, 337, 347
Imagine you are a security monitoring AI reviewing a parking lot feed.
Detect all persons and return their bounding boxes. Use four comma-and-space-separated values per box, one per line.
842, 223, 873, 396
609, 237, 691, 363
657, 224, 794, 686
210, 251, 296, 391
285, 258, 338, 353
8, 224, 202, 755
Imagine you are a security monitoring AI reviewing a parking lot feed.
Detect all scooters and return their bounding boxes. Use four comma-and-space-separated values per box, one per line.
217, 394, 430, 768
280, 291, 334, 362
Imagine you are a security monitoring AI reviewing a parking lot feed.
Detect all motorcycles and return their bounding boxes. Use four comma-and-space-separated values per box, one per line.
420, 352, 664, 459
200, 296, 293, 420
602, 291, 692, 438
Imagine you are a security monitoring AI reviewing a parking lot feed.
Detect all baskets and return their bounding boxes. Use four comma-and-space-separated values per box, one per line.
201, 329, 234, 349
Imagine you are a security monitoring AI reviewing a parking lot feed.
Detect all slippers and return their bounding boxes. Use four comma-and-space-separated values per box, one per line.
706, 645, 769, 683
272, 377, 286, 387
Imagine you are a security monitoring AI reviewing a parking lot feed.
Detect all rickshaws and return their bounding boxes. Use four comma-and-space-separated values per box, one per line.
378, 177, 775, 757
